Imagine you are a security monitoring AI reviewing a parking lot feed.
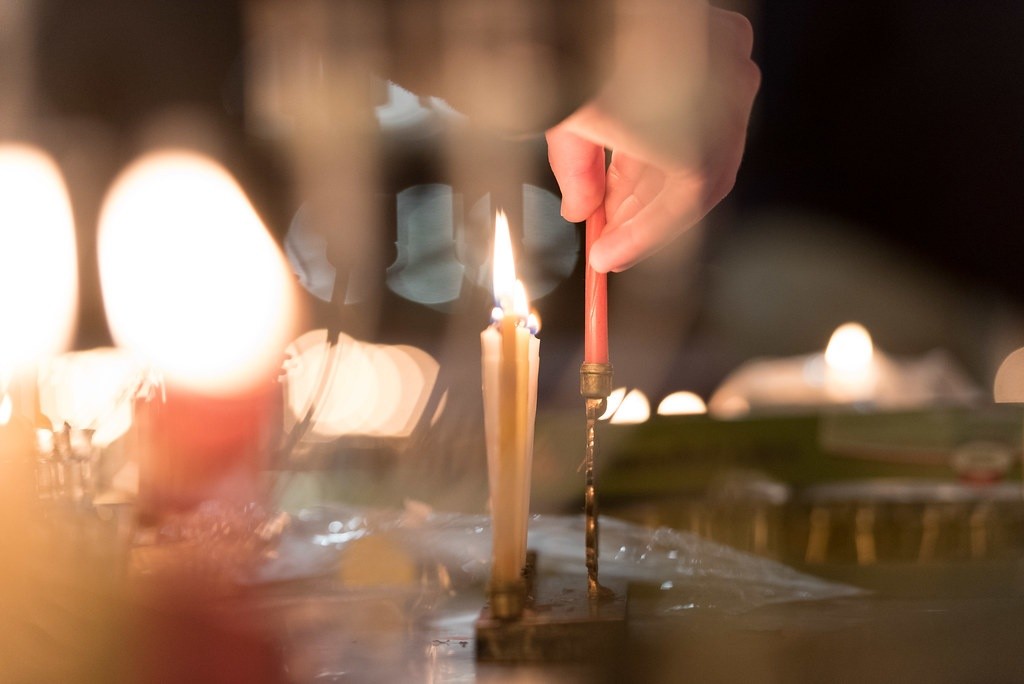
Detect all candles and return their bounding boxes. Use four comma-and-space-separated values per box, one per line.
478, 210, 540, 585
582, 146, 613, 364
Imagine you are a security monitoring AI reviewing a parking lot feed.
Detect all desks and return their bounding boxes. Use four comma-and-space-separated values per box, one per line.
280, 515, 881, 684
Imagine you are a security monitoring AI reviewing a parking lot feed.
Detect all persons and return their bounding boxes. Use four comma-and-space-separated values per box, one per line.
227, 0, 760, 272
